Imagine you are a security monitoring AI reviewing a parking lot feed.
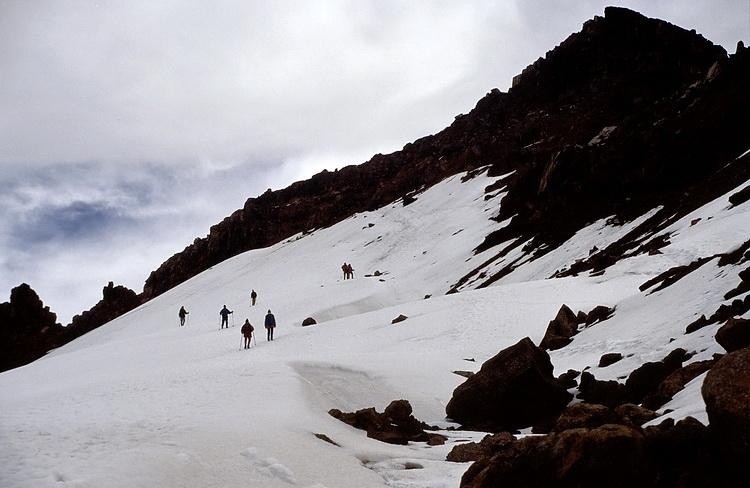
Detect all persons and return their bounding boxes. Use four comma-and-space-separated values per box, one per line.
341, 262, 355, 280
220, 305, 233, 329
178, 306, 189, 326
250, 289, 257, 306
265, 309, 276, 342
240, 319, 254, 349
